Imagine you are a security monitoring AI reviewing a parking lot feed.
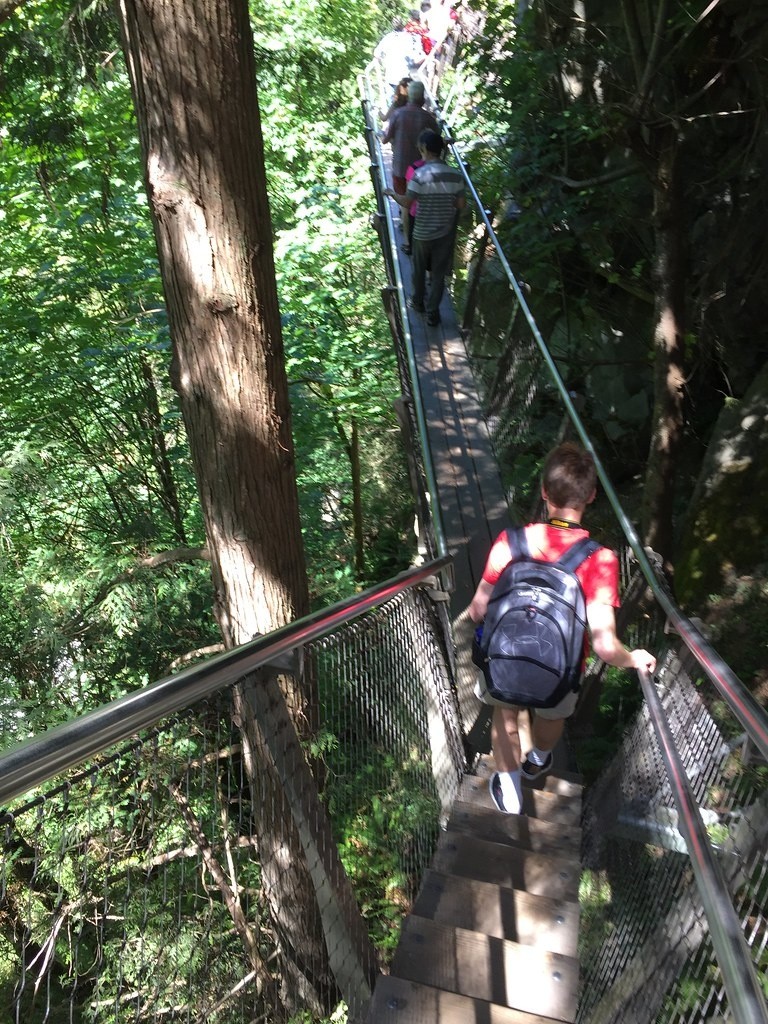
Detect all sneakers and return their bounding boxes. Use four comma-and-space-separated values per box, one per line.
521, 748, 553, 781
489, 771, 508, 814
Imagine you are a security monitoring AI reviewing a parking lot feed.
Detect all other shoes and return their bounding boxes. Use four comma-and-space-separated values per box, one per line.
406, 297, 426, 313
401, 244, 412, 255
398, 223, 403, 231
426, 311, 440, 326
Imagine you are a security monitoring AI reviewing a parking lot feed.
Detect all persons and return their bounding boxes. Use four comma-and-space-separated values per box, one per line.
377, 81, 447, 213
469, 439, 656, 814
401, 157, 448, 255
366, 18, 440, 107
384, 129, 466, 327
404, 0, 455, 56
376, 78, 414, 123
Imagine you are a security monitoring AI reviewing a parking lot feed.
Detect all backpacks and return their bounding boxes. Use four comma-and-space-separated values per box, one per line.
470, 525, 602, 708
